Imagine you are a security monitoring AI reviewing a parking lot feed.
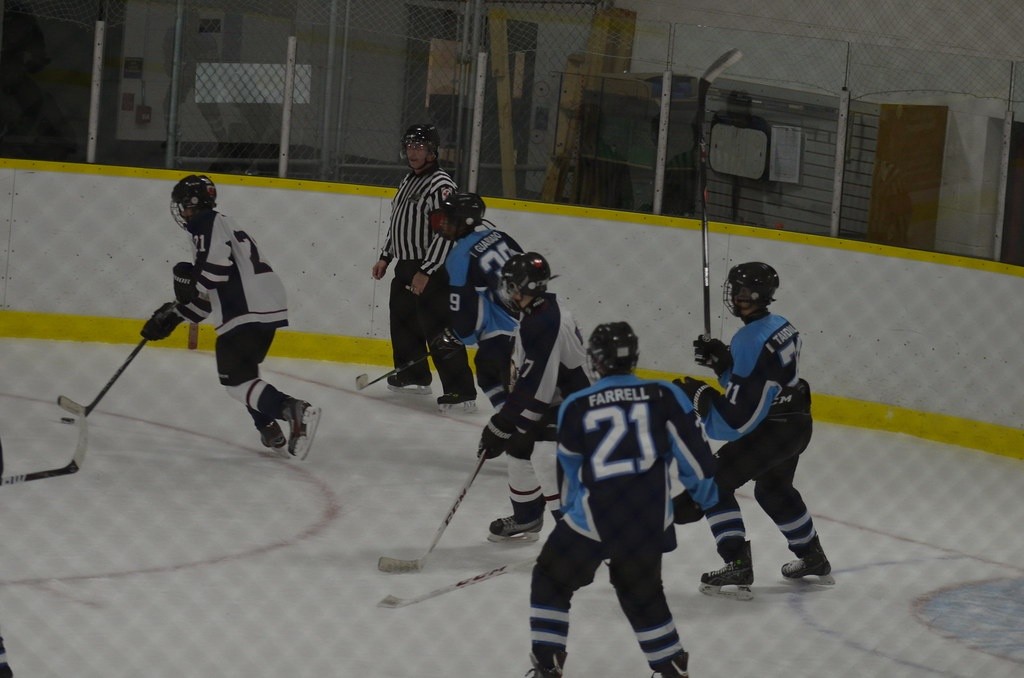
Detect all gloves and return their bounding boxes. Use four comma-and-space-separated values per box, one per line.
431, 329, 462, 357
173, 262, 197, 303
141, 303, 184, 341
670, 490, 704, 523
672, 376, 720, 417
478, 414, 515, 458
694, 336, 727, 369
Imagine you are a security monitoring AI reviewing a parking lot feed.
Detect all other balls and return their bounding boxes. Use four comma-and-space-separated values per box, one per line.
61, 418, 75, 424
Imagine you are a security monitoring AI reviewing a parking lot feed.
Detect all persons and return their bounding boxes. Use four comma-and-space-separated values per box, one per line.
672, 262, 837, 601
439, 192, 526, 415
529, 322, 723, 678
477, 251, 590, 546
141, 175, 322, 461
372, 123, 478, 415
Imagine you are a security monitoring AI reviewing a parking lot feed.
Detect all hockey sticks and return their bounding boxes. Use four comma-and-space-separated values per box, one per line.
696, 45, 746, 338
377, 448, 490, 575
377, 555, 538, 610
356, 348, 440, 392
57, 299, 179, 417
0, 420, 90, 487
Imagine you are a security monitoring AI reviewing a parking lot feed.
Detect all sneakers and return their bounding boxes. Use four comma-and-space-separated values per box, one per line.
387, 370, 434, 394
261, 421, 290, 459
282, 397, 321, 460
437, 387, 479, 411
487, 495, 546, 542
699, 540, 754, 601
781, 538, 834, 586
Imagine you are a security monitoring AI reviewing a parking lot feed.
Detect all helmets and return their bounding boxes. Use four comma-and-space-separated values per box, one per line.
443, 193, 485, 221
586, 322, 640, 374
502, 252, 549, 295
401, 124, 439, 150
170, 175, 216, 230
723, 262, 779, 317
